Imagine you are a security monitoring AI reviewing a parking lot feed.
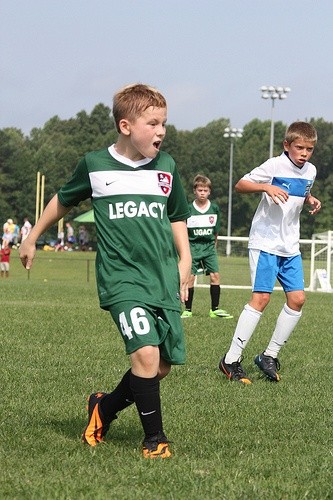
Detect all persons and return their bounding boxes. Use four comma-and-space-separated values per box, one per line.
219, 122, 321, 389
19, 84, 192, 458
0, 218, 88, 279
180, 176, 233, 320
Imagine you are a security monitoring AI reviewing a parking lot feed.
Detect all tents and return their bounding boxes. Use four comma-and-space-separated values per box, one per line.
73, 210, 96, 223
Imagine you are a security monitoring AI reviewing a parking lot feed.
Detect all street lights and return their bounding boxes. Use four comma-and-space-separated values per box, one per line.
260, 85, 291, 159
223, 127, 245, 255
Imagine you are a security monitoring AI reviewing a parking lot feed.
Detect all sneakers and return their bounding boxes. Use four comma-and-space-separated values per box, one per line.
180, 311, 193, 319
82, 392, 110, 448
209, 308, 234, 320
142, 437, 173, 461
254, 351, 281, 382
219, 354, 252, 385
